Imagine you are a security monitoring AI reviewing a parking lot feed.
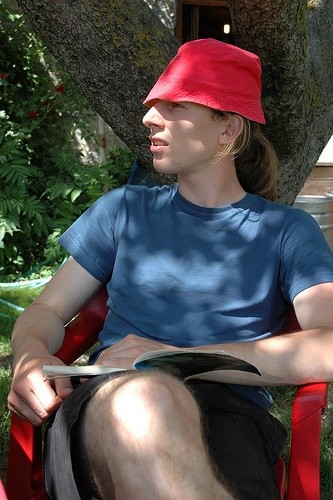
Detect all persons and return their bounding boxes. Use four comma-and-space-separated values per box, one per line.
9, 37, 333, 500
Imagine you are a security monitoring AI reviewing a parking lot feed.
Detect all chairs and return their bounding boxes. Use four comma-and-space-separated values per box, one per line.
5, 285, 328, 500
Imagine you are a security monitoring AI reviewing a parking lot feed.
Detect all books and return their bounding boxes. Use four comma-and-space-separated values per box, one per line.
43, 348, 263, 384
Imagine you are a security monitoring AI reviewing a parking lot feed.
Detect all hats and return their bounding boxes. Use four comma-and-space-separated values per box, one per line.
142, 37, 267, 126
